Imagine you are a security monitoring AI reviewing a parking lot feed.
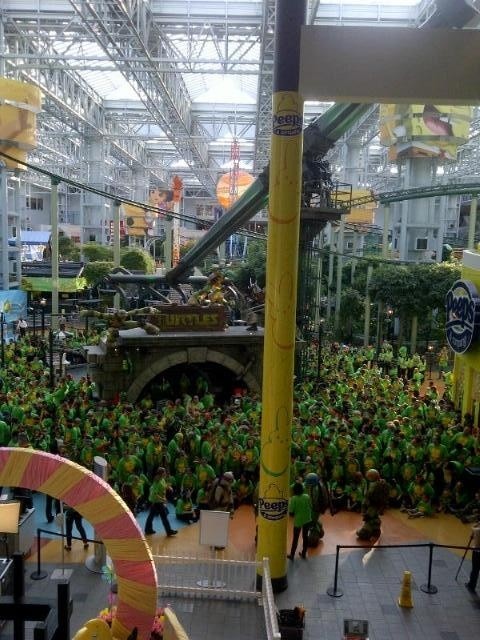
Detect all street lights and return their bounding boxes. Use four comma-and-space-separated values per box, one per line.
39, 297, 47, 339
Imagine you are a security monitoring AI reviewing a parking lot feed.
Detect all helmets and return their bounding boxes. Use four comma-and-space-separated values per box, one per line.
305, 473, 320, 484
366, 468, 380, 480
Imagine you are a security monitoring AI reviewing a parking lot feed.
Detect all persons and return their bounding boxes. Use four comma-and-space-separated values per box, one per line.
0, 326, 479, 540
288, 483, 312, 561
63, 502, 89, 551
16, 317, 27, 336
58, 314, 67, 332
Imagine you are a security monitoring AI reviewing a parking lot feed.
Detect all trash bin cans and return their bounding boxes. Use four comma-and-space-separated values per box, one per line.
343, 618, 369, 640
276, 607, 306, 640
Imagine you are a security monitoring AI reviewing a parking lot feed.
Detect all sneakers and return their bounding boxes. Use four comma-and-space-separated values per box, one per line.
146, 531, 156, 536
165, 530, 177, 536
286, 551, 308, 561
64, 545, 72, 550
82, 543, 88, 549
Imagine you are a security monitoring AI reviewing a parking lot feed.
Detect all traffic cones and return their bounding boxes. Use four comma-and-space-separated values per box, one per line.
398, 571, 415, 607
